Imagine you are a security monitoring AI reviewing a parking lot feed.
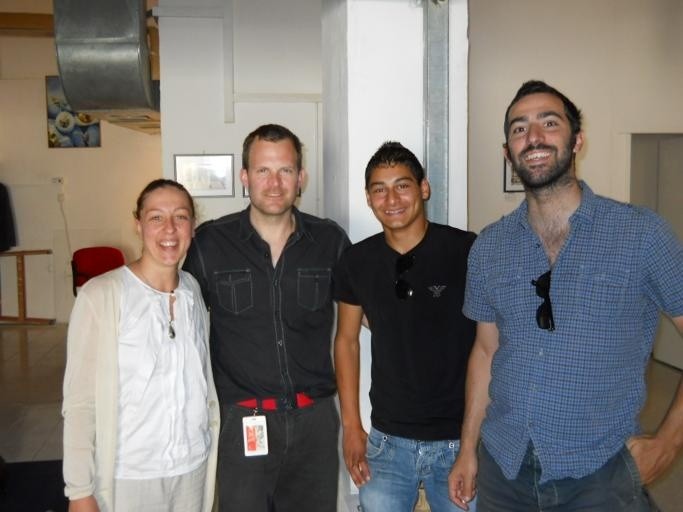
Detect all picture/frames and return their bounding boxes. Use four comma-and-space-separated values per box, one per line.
243, 185, 302, 198
173, 153, 235, 199
44, 75, 102, 148
503, 156, 525, 193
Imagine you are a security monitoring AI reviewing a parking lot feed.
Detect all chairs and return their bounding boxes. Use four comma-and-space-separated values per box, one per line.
70, 246, 127, 296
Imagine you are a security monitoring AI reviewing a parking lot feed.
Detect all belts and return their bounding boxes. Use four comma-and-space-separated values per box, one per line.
236, 392, 315, 410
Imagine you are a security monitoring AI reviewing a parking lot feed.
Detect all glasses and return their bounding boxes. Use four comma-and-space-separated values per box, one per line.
530, 269, 555, 334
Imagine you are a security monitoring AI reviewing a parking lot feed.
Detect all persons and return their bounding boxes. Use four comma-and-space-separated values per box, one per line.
172, 124, 373, 512
444, 76, 683, 511
59, 178, 218, 512
332, 139, 499, 512
